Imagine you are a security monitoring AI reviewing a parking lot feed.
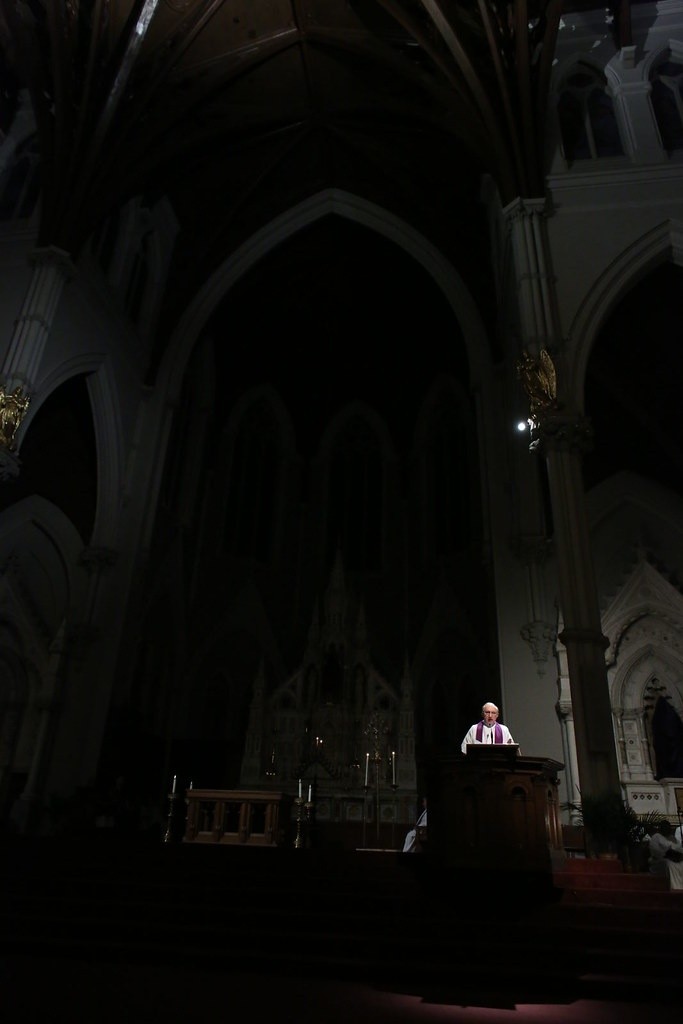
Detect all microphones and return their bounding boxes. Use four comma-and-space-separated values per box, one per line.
488, 724, 494, 744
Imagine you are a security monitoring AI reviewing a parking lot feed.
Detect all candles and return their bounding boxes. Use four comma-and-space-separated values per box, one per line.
316, 737, 319, 746
392, 754, 396, 785
172, 775, 176, 794
308, 785, 311, 802
190, 782, 192, 790
299, 779, 302, 797
365, 756, 368, 788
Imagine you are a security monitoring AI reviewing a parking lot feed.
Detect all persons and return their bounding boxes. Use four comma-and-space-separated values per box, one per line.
401, 794, 427, 853
647, 821, 683, 890
460, 701, 522, 757
